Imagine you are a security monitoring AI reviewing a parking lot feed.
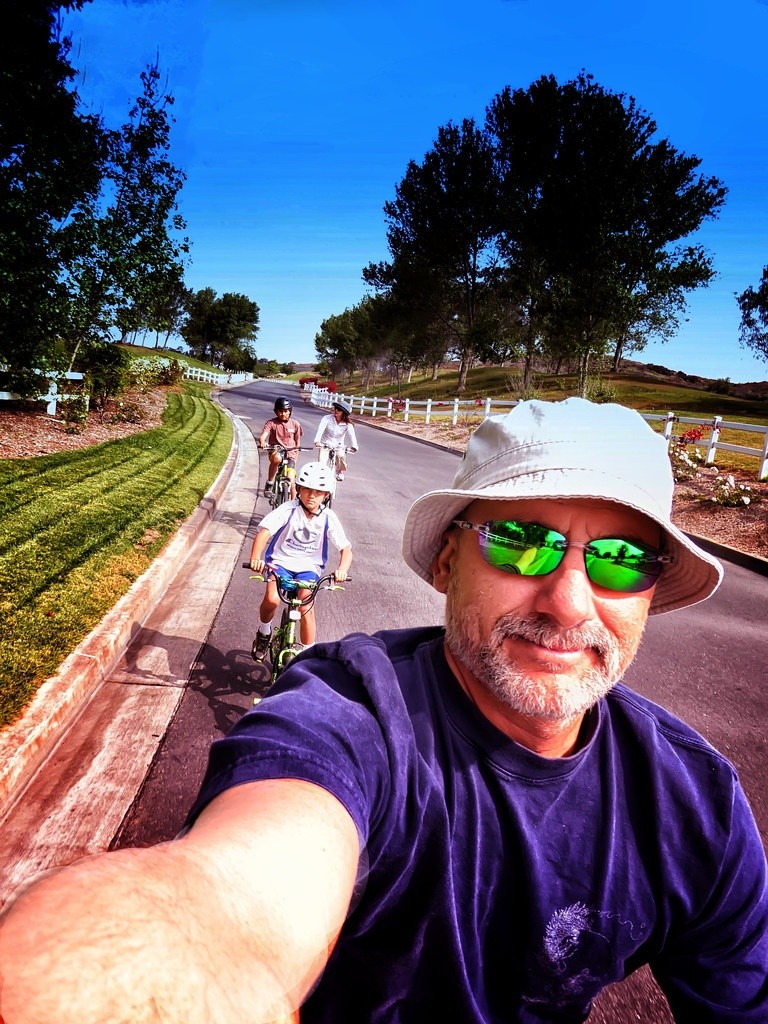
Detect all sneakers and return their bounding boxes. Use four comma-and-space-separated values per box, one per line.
252, 626, 272, 663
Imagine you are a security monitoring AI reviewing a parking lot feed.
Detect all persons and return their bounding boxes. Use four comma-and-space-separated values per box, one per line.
249, 463, 352, 663
314, 401, 359, 481
0, 399, 768, 1024
258, 397, 302, 501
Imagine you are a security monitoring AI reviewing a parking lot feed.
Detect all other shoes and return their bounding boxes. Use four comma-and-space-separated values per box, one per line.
264, 480, 273, 497
336, 470, 344, 480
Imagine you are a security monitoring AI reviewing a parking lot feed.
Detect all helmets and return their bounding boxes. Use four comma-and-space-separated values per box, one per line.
295, 462, 336, 499
274, 396, 293, 412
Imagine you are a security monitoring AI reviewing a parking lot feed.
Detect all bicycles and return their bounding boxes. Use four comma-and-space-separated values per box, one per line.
242, 562, 353, 687
257, 444, 314, 506
320, 443, 355, 510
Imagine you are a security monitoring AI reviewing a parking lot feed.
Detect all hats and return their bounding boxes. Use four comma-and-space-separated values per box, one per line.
332, 401, 352, 415
402, 397, 723, 618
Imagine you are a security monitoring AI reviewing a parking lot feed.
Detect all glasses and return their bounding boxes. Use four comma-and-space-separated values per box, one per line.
335, 406, 343, 411
452, 518, 674, 592
